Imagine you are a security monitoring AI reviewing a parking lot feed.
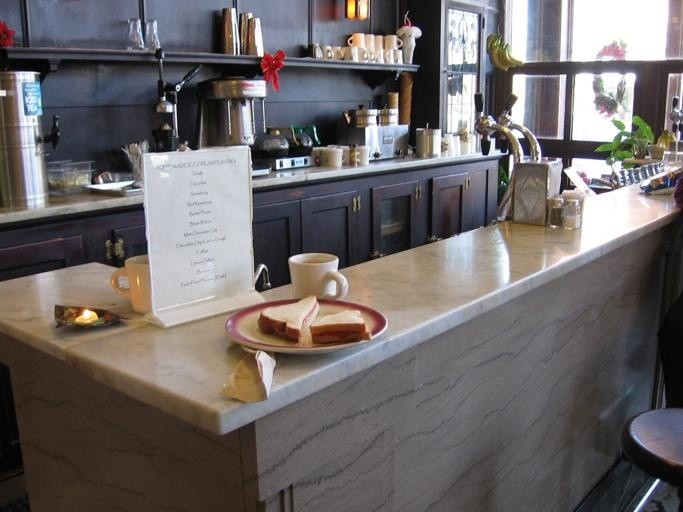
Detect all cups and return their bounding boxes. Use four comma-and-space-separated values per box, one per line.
312, 144, 370, 169
220, 7, 264, 57
442, 134, 496, 157
110, 254, 150, 315
288, 253, 348, 300
123, 18, 144, 51
144, 19, 160, 51
314, 33, 404, 65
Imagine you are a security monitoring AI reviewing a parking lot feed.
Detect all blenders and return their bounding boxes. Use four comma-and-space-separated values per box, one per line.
196, 76, 271, 177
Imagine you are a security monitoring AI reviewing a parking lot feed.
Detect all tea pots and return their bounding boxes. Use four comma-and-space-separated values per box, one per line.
258, 124, 321, 153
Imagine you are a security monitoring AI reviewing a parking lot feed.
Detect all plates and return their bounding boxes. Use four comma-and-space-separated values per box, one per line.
224, 297, 389, 357
85, 179, 144, 198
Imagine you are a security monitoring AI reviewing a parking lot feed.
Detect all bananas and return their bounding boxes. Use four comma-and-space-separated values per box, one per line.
486, 33, 522, 72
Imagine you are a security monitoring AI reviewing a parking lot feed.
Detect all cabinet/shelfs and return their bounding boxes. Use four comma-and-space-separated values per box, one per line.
360, 157, 500, 266
397, 0, 485, 151
84, 179, 361, 293
1, 211, 84, 288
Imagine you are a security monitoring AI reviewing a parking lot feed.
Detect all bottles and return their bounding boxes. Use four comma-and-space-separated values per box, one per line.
548, 198, 563, 229
562, 199, 582, 231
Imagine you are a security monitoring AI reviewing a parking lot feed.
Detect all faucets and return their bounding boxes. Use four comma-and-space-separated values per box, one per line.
476, 113, 542, 220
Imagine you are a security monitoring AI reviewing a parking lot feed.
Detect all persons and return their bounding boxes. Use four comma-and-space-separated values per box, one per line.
657, 176, 683, 409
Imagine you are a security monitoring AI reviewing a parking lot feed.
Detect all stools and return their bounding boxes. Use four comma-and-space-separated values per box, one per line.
619, 406, 682, 512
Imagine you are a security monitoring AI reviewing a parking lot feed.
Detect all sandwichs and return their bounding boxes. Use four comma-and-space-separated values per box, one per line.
258, 294, 319, 343
310, 311, 371, 344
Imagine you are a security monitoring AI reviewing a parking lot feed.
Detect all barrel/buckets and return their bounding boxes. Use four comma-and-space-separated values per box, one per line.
416, 128, 442, 159
